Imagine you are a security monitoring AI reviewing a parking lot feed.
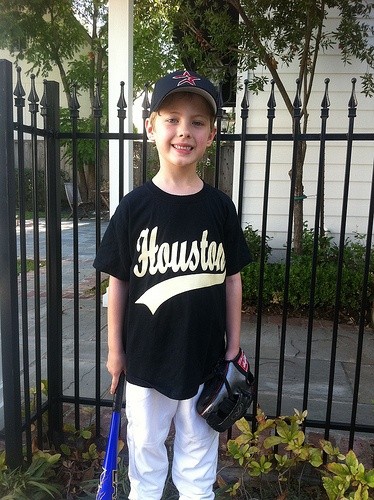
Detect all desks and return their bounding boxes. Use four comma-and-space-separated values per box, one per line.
90, 190, 109, 208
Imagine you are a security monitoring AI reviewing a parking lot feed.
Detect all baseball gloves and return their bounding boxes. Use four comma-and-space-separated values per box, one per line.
196, 347, 256, 434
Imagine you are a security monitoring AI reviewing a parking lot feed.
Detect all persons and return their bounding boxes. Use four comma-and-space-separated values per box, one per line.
92, 72, 252, 500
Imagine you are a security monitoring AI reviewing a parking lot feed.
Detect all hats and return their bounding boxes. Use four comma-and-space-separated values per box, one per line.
150, 70, 220, 114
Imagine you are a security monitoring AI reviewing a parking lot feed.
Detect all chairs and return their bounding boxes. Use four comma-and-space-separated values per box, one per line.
64, 182, 93, 221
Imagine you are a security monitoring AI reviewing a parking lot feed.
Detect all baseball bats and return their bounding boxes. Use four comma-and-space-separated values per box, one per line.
95, 370, 125, 500
